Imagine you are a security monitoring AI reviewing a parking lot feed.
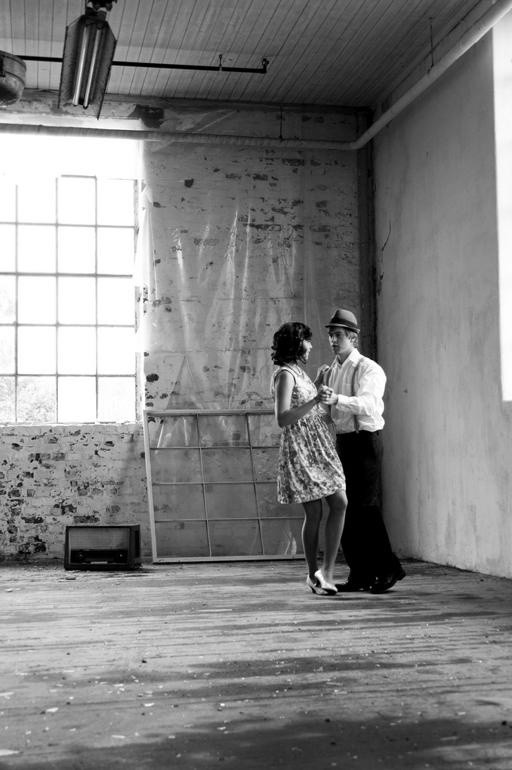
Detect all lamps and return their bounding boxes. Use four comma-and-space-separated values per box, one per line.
0, 51, 26, 106
58, 0, 117, 120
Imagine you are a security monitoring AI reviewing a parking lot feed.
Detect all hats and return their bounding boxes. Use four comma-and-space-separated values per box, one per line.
323, 308, 361, 335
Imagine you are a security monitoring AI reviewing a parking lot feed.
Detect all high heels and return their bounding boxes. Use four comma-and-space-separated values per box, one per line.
305, 568, 339, 596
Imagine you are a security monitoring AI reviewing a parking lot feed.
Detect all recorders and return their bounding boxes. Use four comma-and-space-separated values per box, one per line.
64, 522, 142, 571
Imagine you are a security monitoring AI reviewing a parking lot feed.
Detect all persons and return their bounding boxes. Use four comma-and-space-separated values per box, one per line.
320, 306, 406, 595
268, 321, 349, 596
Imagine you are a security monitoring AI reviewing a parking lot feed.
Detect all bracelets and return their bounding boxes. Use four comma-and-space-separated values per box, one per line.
314, 397, 320, 403
313, 378, 320, 387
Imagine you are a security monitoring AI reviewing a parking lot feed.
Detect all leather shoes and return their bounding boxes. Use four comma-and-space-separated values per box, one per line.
335, 563, 407, 595
